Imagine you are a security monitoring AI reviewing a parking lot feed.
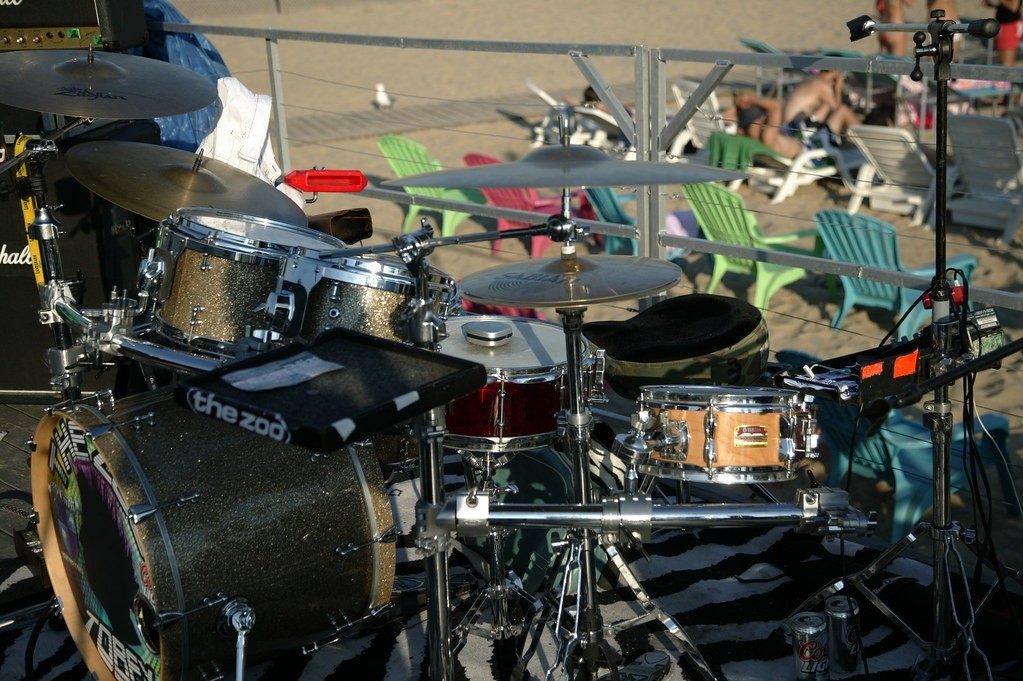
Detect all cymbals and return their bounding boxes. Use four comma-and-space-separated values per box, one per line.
0, 43, 221, 123
63, 137, 310, 233
374, 101, 757, 191
456, 244, 686, 311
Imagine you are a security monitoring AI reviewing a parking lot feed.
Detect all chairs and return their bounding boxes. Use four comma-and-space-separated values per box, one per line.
373, 134, 487, 240
526, 37, 1023, 346
772, 347, 1010, 544
464, 153, 599, 265
461, 292, 542, 327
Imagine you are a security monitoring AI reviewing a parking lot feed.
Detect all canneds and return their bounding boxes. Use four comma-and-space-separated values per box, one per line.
822, 594, 861, 675
789, 612, 830, 681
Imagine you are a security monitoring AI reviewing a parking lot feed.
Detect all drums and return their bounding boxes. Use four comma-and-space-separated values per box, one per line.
29, 378, 401, 681
296, 250, 457, 354
636, 382, 802, 486
437, 310, 597, 456
147, 206, 349, 361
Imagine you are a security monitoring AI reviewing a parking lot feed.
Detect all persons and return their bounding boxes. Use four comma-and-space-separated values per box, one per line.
927, 0, 963, 64
735, 90, 863, 167
874, 0, 913, 56
784, 54, 844, 123
983, 0, 1023, 66
